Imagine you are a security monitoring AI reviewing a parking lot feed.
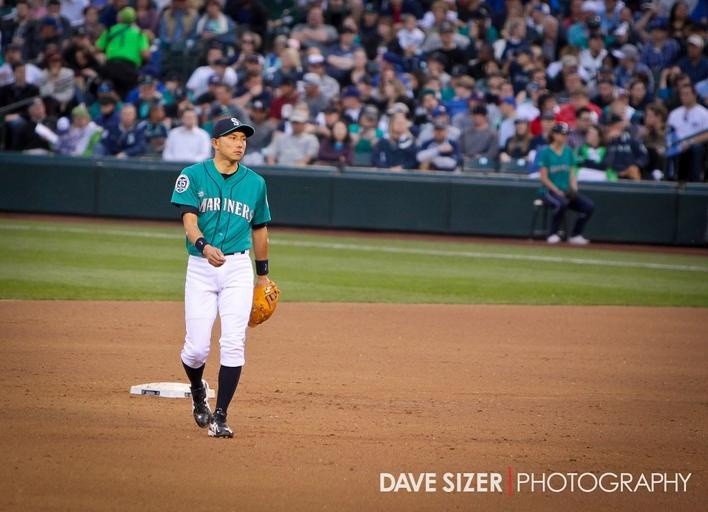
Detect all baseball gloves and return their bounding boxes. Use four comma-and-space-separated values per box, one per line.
249, 278, 280, 327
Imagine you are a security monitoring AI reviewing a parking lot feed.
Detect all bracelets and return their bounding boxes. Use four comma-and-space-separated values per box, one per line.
256, 259, 269, 275
195, 238, 210, 255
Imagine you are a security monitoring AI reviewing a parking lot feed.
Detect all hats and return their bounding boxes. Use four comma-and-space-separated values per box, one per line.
686, 33, 704, 48
423, 89, 448, 129
553, 121, 574, 134
307, 54, 325, 64
290, 112, 307, 123
387, 102, 410, 115
611, 43, 640, 62
303, 72, 320, 85
211, 117, 255, 139
208, 53, 259, 85
439, 21, 455, 32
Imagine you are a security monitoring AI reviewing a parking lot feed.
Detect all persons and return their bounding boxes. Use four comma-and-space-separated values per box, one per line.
534, 122, 595, 244
170, 117, 279, 439
1, 0, 706, 183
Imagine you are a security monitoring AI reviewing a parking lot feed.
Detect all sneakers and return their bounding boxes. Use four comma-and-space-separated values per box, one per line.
190, 379, 211, 428
548, 234, 590, 247
207, 407, 233, 438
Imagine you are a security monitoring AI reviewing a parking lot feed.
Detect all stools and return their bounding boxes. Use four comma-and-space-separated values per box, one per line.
527, 199, 567, 242
